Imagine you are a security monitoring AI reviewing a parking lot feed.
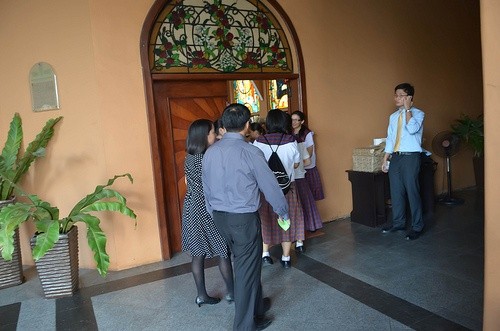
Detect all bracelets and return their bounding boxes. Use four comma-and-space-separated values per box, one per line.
405, 110, 411, 113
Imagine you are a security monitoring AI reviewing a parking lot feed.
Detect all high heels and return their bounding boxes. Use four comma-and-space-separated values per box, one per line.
226, 295, 234, 304
196, 295, 221, 307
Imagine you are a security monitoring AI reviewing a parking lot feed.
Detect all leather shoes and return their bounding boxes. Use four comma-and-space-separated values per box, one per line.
405, 231, 422, 240
382, 226, 406, 233
280, 259, 291, 268
263, 257, 274, 265
295, 245, 304, 252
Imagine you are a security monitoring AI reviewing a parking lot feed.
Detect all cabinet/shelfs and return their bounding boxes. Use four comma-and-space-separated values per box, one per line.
345, 158, 438, 227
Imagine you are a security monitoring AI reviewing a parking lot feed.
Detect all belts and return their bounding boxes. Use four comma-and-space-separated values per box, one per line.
394, 152, 419, 156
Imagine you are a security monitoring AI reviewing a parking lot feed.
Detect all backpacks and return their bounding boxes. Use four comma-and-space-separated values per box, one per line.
263, 134, 292, 195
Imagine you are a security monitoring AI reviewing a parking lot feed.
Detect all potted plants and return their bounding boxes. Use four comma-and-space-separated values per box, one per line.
450, 111, 484, 192
0, 113, 64, 290
0, 170, 137, 299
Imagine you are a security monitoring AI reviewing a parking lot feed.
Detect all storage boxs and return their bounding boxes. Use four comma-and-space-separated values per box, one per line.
353, 147, 384, 172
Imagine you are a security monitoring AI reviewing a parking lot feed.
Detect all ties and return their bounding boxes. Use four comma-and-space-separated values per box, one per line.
393, 112, 403, 152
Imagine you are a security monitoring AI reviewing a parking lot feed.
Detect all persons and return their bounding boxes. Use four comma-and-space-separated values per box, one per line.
213, 118, 228, 144
201, 103, 290, 331
181, 119, 234, 307
245, 108, 325, 268
379, 82, 425, 241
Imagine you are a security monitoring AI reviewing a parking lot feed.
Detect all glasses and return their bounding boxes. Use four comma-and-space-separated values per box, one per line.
393, 94, 408, 99
292, 120, 301, 122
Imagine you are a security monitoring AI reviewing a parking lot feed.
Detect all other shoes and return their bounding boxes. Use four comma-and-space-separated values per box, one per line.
263, 298, 270, 312
259, 318, 271, 330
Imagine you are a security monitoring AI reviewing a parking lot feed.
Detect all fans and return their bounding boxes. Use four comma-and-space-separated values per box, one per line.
432, 130, 464, 206
